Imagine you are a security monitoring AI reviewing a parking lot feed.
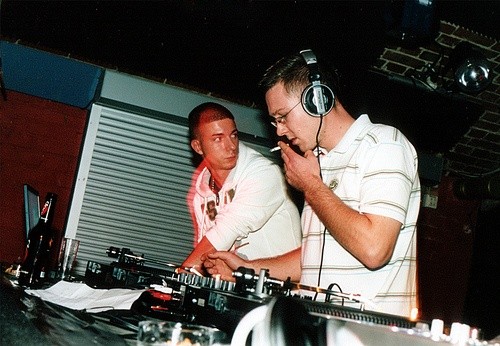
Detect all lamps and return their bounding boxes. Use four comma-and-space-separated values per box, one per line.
450, 46, 497, 96
384, 0, 440, 50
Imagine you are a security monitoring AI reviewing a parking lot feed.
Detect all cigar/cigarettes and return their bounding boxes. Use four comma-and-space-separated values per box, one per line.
269, 144, 290, 153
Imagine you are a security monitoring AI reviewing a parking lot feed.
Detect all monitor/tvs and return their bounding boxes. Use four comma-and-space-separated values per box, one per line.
23, 184, 41, 239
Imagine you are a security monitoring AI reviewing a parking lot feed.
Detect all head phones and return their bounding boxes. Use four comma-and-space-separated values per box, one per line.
299, 48, 335, 117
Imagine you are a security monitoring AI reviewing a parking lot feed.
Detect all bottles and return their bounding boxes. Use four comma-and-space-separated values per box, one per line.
18, 192, 58, 289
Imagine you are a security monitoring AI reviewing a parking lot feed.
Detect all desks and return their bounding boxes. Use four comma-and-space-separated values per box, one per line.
1, 259, 233, 345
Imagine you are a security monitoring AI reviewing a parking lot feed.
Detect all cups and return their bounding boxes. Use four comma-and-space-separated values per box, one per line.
53, 238, 80, 284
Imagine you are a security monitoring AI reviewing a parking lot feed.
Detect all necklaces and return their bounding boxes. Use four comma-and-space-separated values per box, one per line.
210, 177, 216, 191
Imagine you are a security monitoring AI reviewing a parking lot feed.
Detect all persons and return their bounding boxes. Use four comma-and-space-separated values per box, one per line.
181, 102, 301, 282
201, 53, 422, 322
